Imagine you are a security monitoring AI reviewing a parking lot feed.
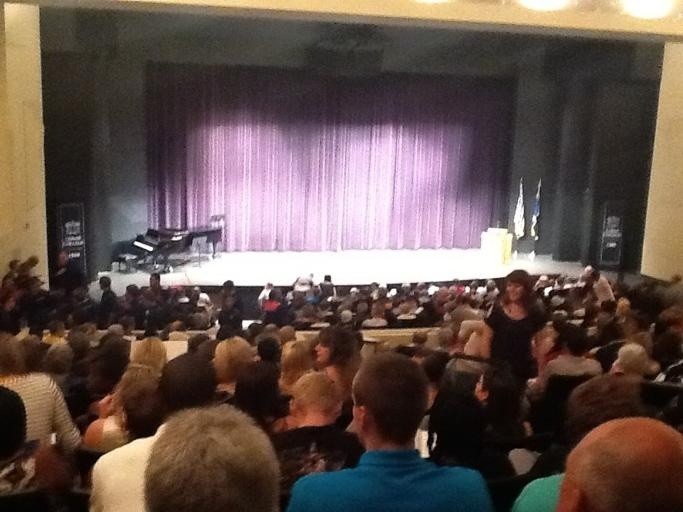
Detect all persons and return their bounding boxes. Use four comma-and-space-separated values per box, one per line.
1, 250, 683, 512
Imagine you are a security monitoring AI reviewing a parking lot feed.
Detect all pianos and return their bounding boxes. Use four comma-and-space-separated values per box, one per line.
130, 221, 222, 274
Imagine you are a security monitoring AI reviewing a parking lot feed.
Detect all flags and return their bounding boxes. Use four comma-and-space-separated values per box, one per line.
530, 182, 542, 241
513, 178, 526, 241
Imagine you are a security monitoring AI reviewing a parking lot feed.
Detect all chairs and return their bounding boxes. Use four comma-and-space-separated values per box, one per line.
0, 285, 682, 512
